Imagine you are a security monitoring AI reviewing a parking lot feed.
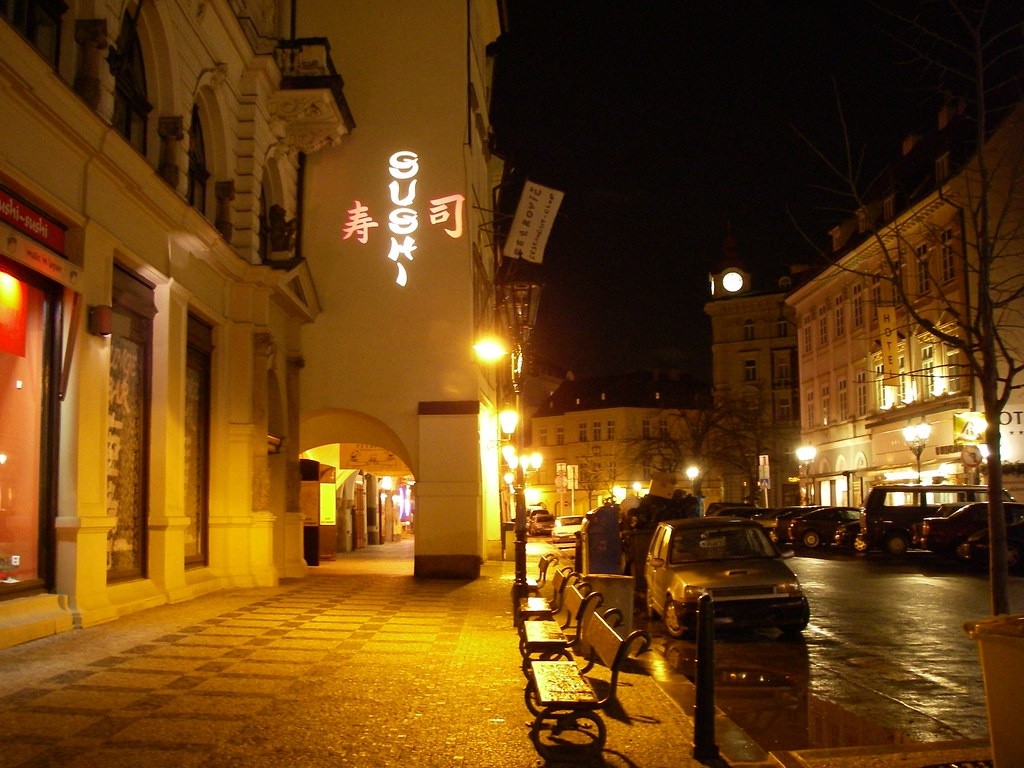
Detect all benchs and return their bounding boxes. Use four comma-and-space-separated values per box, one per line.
510, 553, 652, 762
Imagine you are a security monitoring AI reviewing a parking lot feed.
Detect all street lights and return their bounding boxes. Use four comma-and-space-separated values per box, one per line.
685, 465, 699, 496
496, 402, 544, 629
633, 482, 641, 497
901, 422, 933, 484
795, 445, 817, 507
473, 335, 508, 561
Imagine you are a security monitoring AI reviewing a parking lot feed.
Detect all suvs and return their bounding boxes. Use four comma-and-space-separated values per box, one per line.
707, 506, 782, 547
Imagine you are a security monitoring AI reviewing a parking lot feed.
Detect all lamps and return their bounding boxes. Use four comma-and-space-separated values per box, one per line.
497, 409, 543, 495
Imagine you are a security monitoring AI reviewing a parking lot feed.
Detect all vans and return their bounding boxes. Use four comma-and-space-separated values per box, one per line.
529, 510, 550, 519
858, 484, 1016, 558
705, 501, 751, 515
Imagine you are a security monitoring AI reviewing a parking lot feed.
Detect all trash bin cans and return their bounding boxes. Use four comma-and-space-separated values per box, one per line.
573, 530, 583, 573
580, 503, 625, 575
963, 613, 1023, 768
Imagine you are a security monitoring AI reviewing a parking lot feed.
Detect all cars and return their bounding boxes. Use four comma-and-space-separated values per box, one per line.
641, 515, 811, 641
527, 508, 555, 537
828, 521, 867, 553
958, 517, 1024, 581
787, 507, 866, 549
916, 500, 1024, 555
550, 515, 586, 544
911, 501, 974, 551
